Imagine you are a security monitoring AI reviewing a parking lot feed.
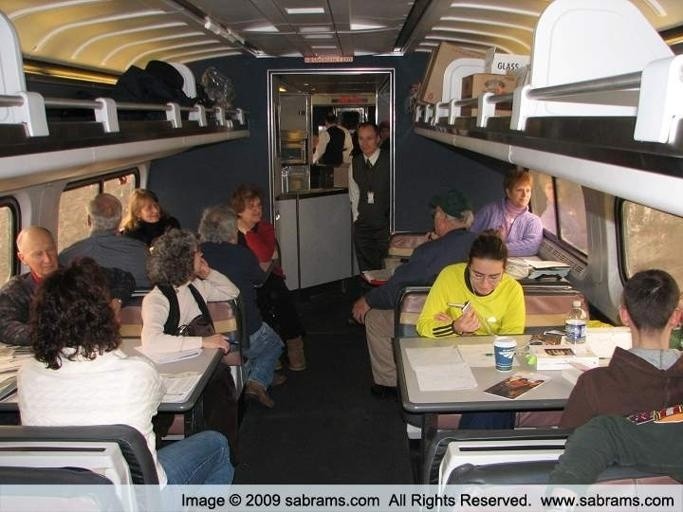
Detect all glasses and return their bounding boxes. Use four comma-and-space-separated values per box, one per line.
466, 264, 504, 286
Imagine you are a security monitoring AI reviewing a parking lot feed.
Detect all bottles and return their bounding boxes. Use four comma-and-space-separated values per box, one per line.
565, 300, 587, 355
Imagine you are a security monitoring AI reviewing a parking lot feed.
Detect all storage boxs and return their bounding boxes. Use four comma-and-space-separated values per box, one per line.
461, 73, 516, 118
415, 40, 485, 107
485, 45, 530, 75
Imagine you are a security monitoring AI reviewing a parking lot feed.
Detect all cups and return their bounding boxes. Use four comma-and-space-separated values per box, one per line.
494, 337, 517, 374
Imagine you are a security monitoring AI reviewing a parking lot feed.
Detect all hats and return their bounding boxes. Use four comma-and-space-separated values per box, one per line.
431, 188, 474, 219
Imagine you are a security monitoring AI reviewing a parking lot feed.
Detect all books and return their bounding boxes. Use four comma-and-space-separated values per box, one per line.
483, 370, 552, 400
529, 344, 600, 385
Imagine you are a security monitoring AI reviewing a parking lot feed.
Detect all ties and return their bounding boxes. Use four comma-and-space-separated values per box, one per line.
364, 160, 372, 169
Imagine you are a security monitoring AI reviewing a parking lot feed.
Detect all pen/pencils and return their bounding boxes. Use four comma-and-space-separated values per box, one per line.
226, 340, 240, 344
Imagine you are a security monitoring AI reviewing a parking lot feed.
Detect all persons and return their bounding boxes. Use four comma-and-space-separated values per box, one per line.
57, 112, 546, 409
0, 226, 135, 347
141, 228, 241, 467
16, 264, 235, 512
415, 236, 527, 429
546, 404, 683, 512
558, 270, 683, 429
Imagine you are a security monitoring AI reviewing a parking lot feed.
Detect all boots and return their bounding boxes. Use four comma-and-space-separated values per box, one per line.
285, 334, 307, 372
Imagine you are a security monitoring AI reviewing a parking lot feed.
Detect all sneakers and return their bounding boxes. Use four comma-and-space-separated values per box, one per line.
371, 384, 392, 400
243, 380, 276, 409
